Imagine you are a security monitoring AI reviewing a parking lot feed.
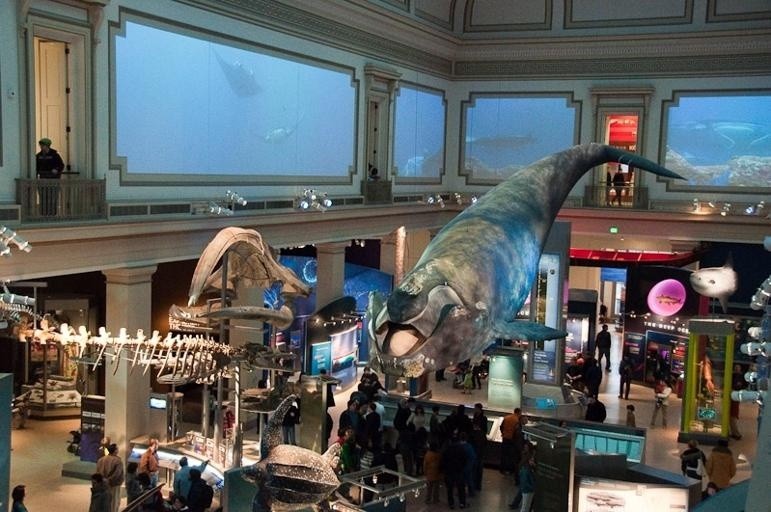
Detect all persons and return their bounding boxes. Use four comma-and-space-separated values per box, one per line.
321, 365, 534, 512
12, 485, 27, 512
257, 381, 298, 445
612, 168, 626, 206
565, 304, 668, 427
607, 167, 612, 206
681, 357, 745, 492
89, 440, 213, 512
36, 138, 65, 216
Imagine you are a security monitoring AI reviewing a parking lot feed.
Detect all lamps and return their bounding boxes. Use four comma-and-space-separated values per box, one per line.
308, 308, 365, 330
293, 188, 334, 214
730, 276, 770, 405
203, 187, 249, 218
0, 223, 34, 259
1, 288, 37, 312
424, 191, 479, 209
692, 193, 768, 218
